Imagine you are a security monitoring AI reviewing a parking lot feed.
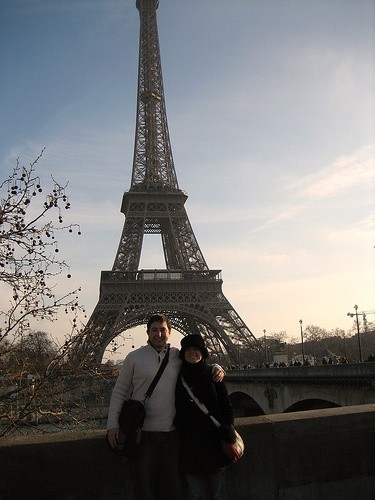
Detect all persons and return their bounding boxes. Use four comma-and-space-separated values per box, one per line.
107, 315, 226, 500
222, 359, 310, 370
322, 355, 347, 364
368, 352, 375, 362
173, 334, 240, 500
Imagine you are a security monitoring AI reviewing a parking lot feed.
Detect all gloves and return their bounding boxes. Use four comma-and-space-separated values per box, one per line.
225, 443, 240, 464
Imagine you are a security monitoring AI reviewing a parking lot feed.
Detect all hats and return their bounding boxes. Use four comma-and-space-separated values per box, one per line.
179, 335, 209, 361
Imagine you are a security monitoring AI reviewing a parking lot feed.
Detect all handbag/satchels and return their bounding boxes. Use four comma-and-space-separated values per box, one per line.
222, 430, 244, 464
119, 401, 145, 430
